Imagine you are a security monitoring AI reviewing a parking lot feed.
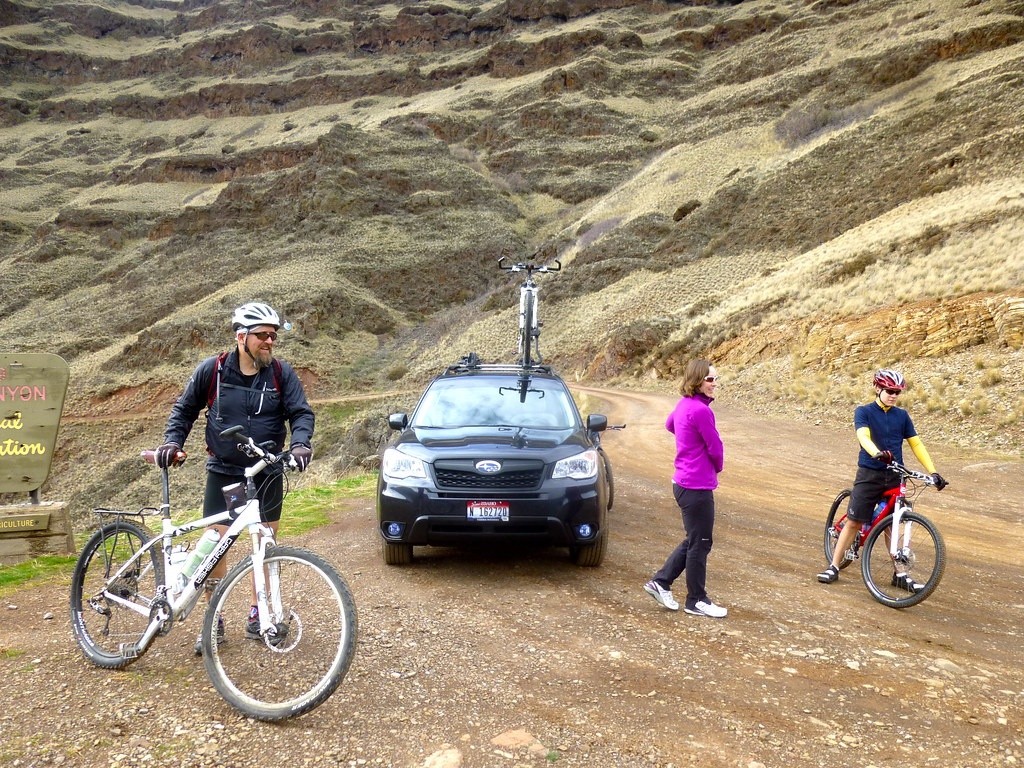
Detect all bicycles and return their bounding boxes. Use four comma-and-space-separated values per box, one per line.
499, 373, 545, 403
823, 454, 950, 609
69, 424, 358, 722
585, 424, 626, 510
498, 253, 561, 369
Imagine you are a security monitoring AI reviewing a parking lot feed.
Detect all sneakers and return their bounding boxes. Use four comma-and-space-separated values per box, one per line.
643, 580, 679, 609
683, 602, 728, 617
244, 617, 281, 644
194, 618, 224, 655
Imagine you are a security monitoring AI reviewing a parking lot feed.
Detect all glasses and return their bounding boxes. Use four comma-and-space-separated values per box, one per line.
247, 331, 277, 342
703, 376, 717, 382
882, 388, 901, 395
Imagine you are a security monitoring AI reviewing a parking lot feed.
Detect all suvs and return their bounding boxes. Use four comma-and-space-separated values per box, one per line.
377, 352, 607, 566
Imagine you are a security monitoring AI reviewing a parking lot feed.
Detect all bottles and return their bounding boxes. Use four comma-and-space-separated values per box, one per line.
170, 542, 190, 597
181, 528, 220, 578
865, 502, 887, 530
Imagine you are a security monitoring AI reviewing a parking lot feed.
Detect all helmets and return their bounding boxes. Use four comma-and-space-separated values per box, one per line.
873, 368, 905, 390
233, 303, 280, 332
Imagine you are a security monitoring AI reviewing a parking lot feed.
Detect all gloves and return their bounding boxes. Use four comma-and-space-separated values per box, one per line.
154, 441, 186, 469
877, 450, 894, 462
287, 444, 313, 471
931, 472, 944, 490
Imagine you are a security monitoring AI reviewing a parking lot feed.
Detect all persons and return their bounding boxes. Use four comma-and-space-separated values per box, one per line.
643, 359, 726, 619
154, 303, 315, 654
817, 367, 945, 592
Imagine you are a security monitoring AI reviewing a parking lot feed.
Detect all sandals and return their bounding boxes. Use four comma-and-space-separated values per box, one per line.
818, 565, 839, 584
890, 573, 922, 592
